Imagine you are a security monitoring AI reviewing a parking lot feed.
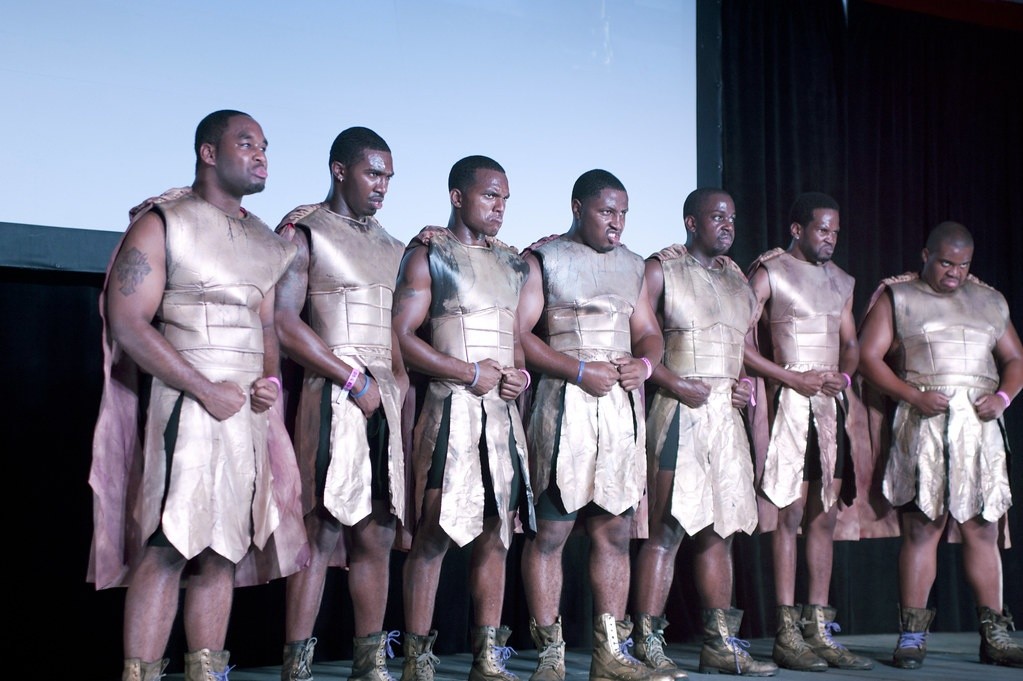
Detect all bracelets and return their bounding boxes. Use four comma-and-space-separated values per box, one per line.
467, 362, 479, 388
350, 375, 369, 398
337, 368, 360, 404
270, 376, 281, 391
997, 391, 1010, 408
841, 372, 852, 388
576, 360, 584, 386
521, 369, 531, 391
641, 357, 651, 379
740, 377, 756, 406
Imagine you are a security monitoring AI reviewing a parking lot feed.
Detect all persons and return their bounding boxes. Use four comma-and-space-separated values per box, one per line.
274, 127, 405, 681
87, 109, 311, 681
640, 189, 779, 681
518, 170, 672, 681
859, 221, 1023, 667
744, 194, 876, 671
394, 155, 538, 681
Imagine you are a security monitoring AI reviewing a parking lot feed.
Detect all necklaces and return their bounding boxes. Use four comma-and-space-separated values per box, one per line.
446, 227, 460, 243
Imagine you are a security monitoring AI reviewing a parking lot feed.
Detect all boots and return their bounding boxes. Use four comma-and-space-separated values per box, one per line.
281, 637, 318, 681
698, 607, 780, 677
468, 625, 523, 681
399, 630, 440, 681
346, 630, 401, 681
588, 613, 675, 681
976, 604, 1023, 669
528, 615, 566, 681
893, 607, 937, 670
633, 613, 690, 681
771, 605, 828, 671
800, 604, 874, 670
184, 648, 237, 681
121, 657, 170, 681
